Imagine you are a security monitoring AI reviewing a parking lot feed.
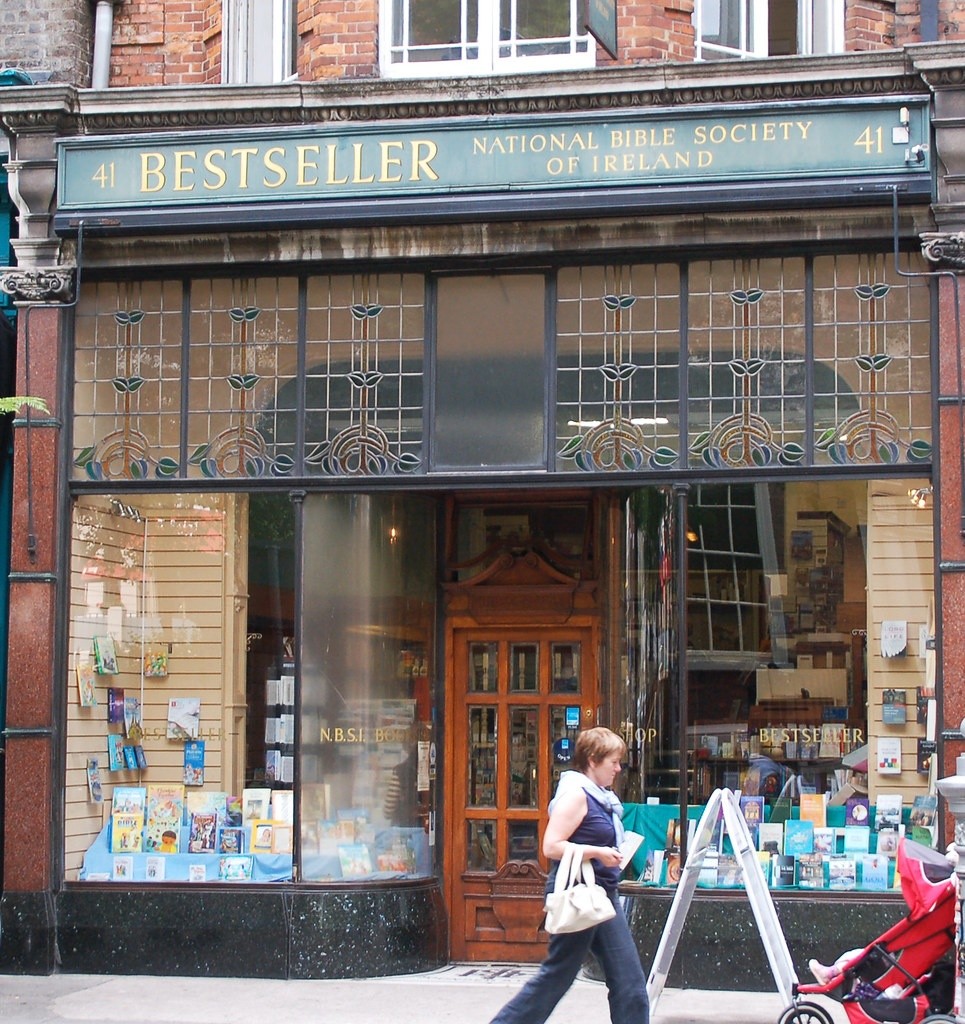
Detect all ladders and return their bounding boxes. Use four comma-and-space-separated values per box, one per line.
643, 788, 804, 1014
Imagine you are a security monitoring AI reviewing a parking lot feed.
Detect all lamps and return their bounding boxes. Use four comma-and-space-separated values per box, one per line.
905, 486, 935, 509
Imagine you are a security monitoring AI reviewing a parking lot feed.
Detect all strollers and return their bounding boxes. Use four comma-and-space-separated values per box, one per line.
777, 836, 965, 1024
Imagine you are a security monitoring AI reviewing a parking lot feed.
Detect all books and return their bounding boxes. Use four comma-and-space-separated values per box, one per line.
123, 745, 138, 770
87, 760, 98, 770
167, 698, 200, 740
124, 697, 140, 739
183, 741, 205, 786
881, 621, 908, 659
88, 769, 103, 803
882, 689, 907, 725
134, 746, 147, 768
95, 635, 117, 674
85, 785, 416, 882
916, 686, 936, 723
144, 650, 167, 677
639, 739, 937, 889
107, 688, 125, 724
108, 735, 125, 771
77, 666, 97, 706
919, 625, 926, 667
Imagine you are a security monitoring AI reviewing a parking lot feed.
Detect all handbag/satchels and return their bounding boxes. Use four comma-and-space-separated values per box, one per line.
543, 843, 617, 935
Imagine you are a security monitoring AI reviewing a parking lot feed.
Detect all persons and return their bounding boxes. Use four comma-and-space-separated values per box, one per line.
809, 948, 865, 987
490, 727, 649, 1023
384, 721, 430, 832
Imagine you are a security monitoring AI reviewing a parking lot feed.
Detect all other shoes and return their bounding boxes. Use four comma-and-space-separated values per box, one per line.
809, 960, 832, 985
